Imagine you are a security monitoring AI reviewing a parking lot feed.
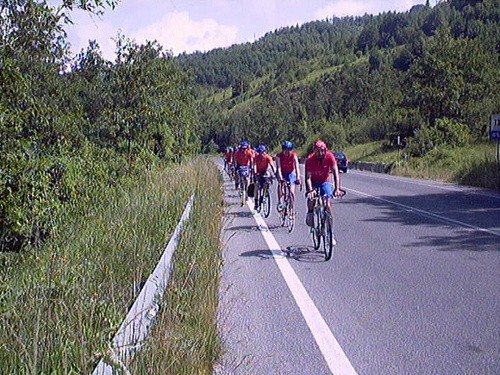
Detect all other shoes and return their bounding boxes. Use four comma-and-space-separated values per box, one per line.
259, 196, 263, 203
253, 203, 258, 210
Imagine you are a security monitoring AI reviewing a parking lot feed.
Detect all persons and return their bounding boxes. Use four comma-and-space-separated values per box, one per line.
253, 145, 276, 210
225, 142, 256, 201
276, 141, 302, 220
305, 140, 343, 246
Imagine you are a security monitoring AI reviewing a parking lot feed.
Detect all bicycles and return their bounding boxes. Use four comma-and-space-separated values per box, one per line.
305, 189, 346, 261
276, 178, 303, 233
223, 158, 277, 219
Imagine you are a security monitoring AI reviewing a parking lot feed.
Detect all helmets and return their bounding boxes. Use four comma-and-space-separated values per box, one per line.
241, 142, 248, 148
282, 141, 293, 151
257, 146, 266, 153
225, 145, 241, 153
252, 147, 259, 153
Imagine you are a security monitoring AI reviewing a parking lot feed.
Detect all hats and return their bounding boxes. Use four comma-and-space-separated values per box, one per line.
313, 140, 326, 153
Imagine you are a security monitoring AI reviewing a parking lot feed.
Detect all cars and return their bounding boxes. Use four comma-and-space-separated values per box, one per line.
330, 152, 349, 173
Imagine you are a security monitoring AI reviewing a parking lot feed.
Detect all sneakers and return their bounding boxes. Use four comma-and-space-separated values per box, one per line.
327, 232, 336, 246
305, 213, 315, 227
